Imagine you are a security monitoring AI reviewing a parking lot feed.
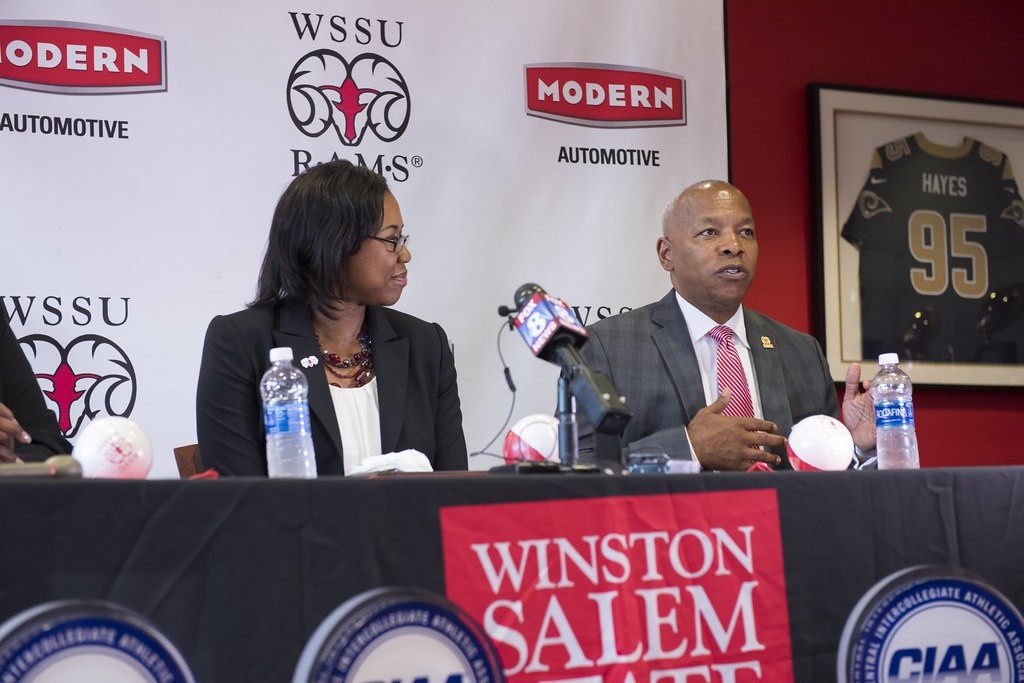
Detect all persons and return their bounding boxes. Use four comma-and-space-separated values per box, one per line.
554, 179, 880, 476
195, 159, 469, 477
0, 296, 75, 469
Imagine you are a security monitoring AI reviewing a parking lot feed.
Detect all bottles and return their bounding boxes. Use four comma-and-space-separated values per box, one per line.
871, 353, 919, 470
260, 347, 317, 479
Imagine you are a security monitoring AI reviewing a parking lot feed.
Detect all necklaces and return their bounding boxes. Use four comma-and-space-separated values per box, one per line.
315, 332, 376, 389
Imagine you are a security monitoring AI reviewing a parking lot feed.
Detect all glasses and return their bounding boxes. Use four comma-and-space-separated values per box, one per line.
369, 232, 410, 253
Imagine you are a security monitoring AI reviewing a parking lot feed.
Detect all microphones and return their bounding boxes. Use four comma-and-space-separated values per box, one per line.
0, 454, 82, 478
513, 284, 634, 434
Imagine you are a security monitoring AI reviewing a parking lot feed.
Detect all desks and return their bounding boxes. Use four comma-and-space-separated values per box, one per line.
0, 467, 1024, 683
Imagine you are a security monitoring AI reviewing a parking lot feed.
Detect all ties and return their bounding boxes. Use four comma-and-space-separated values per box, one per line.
707, 326, 760, 474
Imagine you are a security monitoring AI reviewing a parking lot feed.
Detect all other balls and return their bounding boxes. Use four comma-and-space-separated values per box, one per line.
503, 413, 564, 466
70, 415, 155, 479
786, 414, 855, 472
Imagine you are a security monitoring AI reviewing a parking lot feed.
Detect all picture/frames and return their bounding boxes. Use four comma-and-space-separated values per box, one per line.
807, 79, 1024, 388
